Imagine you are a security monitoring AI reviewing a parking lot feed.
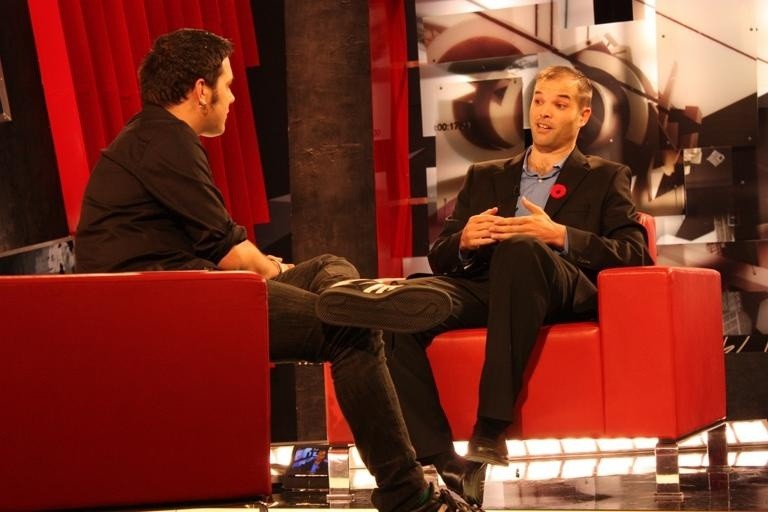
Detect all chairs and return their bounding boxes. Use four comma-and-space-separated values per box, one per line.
324, 212, 729, 503
0, 271, 272, 510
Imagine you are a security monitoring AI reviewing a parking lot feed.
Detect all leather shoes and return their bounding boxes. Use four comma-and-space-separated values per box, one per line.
463, 425, 508, 466
441, 456, 486, 508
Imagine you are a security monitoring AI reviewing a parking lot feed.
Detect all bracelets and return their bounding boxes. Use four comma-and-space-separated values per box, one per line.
275, 259, 284, 274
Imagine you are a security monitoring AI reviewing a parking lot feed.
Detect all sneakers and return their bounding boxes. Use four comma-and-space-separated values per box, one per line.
404, 482, 484, 512
315, 279, 452, 333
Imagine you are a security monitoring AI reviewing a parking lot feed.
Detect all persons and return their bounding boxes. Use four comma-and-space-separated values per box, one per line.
73, 29, 484, 512
294, 447, 327, 473
381, 66, 654, 505
